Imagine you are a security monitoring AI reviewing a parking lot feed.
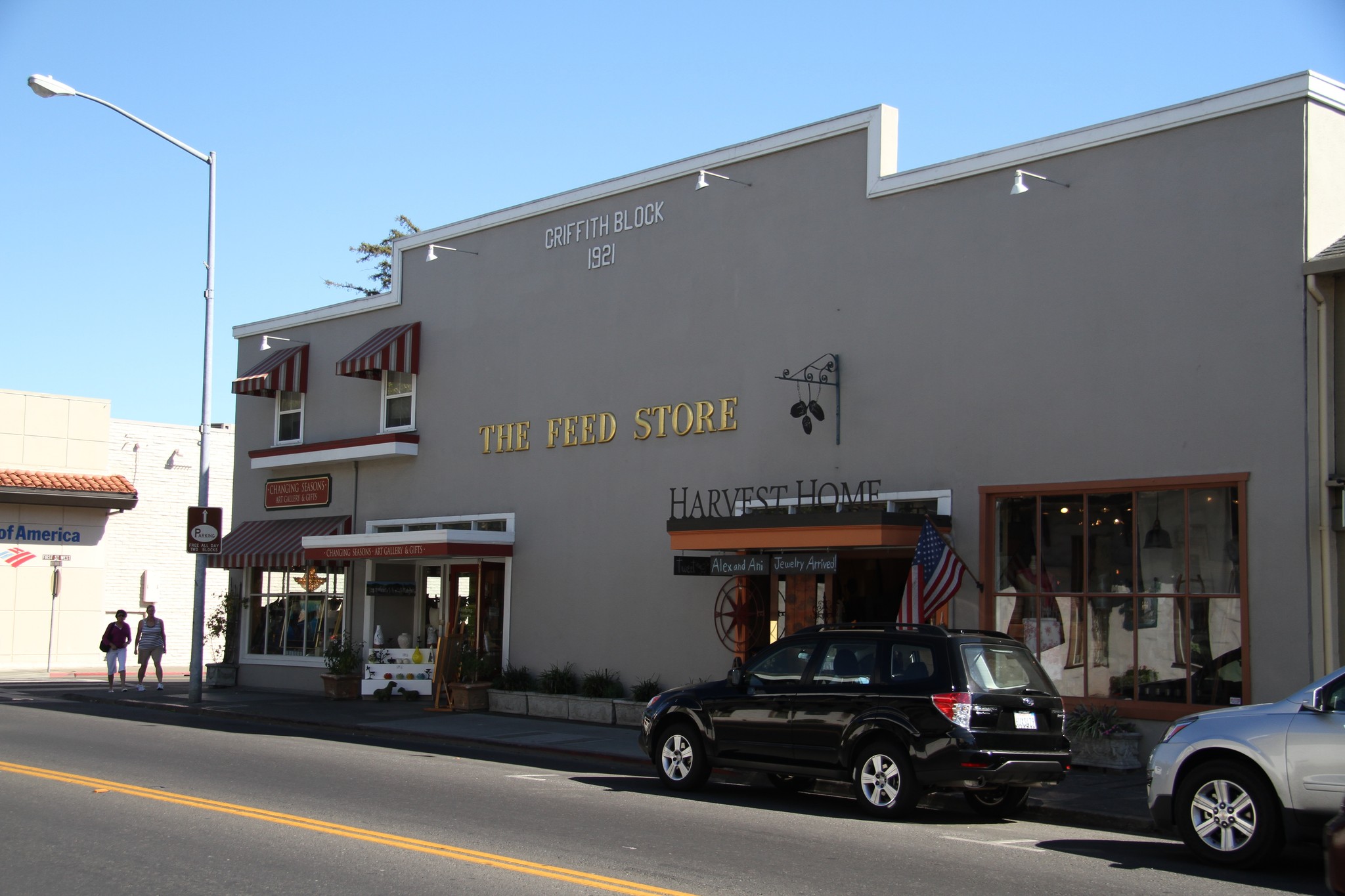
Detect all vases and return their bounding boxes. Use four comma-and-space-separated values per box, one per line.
368, 649, 377, 664
428, 645, 435, 664
373, 625, 384, 648
412, 646, 423, 664
427, 627, 438, 649
397, 633, 412, 649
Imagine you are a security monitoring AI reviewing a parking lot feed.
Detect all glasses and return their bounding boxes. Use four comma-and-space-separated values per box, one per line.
117, 615, 126, 617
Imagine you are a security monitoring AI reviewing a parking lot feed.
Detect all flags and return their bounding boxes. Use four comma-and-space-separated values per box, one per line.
896, 518, 966, 630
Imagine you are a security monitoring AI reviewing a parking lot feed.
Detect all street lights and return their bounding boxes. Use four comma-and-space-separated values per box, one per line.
28, 69, 221, 711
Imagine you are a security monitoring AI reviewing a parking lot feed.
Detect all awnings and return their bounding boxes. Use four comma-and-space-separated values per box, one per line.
205, 514, 351, 569
336, 322, 421, 381
231, 345, 309, 398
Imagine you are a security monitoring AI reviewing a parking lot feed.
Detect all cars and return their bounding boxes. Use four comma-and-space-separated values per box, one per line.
1144, 665, 1345, 868
1134, 646, 1243, 704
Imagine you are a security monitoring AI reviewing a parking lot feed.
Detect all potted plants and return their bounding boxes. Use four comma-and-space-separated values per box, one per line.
202, 589, 249, 688
446, 640, 662, 726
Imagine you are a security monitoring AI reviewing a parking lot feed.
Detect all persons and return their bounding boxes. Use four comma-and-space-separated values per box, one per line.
1016, 555, 1056, 617
134, 605, 166, 691
102, 609, 132, 693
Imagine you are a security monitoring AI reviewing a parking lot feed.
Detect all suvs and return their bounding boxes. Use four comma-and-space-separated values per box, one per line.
635, 619, 1075, 821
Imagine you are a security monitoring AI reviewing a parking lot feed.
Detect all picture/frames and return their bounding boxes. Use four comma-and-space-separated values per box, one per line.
278, 592, 327, 650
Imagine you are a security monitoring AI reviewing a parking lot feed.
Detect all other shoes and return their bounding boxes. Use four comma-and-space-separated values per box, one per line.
121, 689, 128, 691
108, 689, 114, 693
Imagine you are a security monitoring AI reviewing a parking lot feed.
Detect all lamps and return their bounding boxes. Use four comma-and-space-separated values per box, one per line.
694, 170, 752, 191
1142, 493, 1174, 550
1009, 169, 1070, 195
259, 335, 310, 351
292, 566, 327, 592
426, 244, 479, 262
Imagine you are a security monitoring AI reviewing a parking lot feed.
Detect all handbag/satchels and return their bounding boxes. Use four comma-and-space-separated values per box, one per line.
100, 622, 115, 653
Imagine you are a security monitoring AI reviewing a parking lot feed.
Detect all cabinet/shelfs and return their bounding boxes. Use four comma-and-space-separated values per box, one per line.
361, 647, 438, 701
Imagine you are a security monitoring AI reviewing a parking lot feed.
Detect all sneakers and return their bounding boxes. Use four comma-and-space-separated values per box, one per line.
136, 684, 146, 691
157, 683, 164, 690
